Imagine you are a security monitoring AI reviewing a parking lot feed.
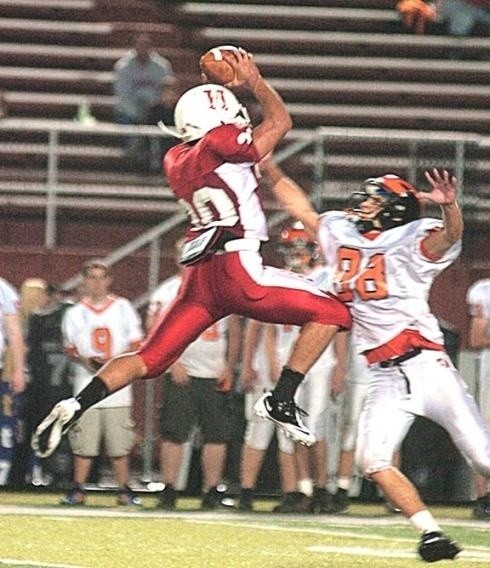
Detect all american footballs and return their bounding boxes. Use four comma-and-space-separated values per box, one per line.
200, 46, 249, 86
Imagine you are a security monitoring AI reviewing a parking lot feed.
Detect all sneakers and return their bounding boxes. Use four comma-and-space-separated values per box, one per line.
118, 489, 142, 507
159, 489, 175, 510
475, 492, 490, 518
31, 398, 82, 458
238, 489, 253, 510
59, 489, 85, 504
272, 487, 349, 513
255, 392, 316, 448
201, 487, 233, 509
418, 531, 461, 563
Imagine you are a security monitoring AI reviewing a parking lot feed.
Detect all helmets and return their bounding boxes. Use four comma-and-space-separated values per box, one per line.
174, 83, 250, 144
345, 174, 424, 234
275, 221, 321, 266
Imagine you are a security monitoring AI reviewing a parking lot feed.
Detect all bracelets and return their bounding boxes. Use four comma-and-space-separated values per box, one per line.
253, 74, 262, 95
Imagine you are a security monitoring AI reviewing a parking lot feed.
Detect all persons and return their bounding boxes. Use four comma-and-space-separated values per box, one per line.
0, 221, 490, 519
111, 34, 180, 174
30, 46, 352, 457
254, 150, 490, 562
396, 0, 489, 36
